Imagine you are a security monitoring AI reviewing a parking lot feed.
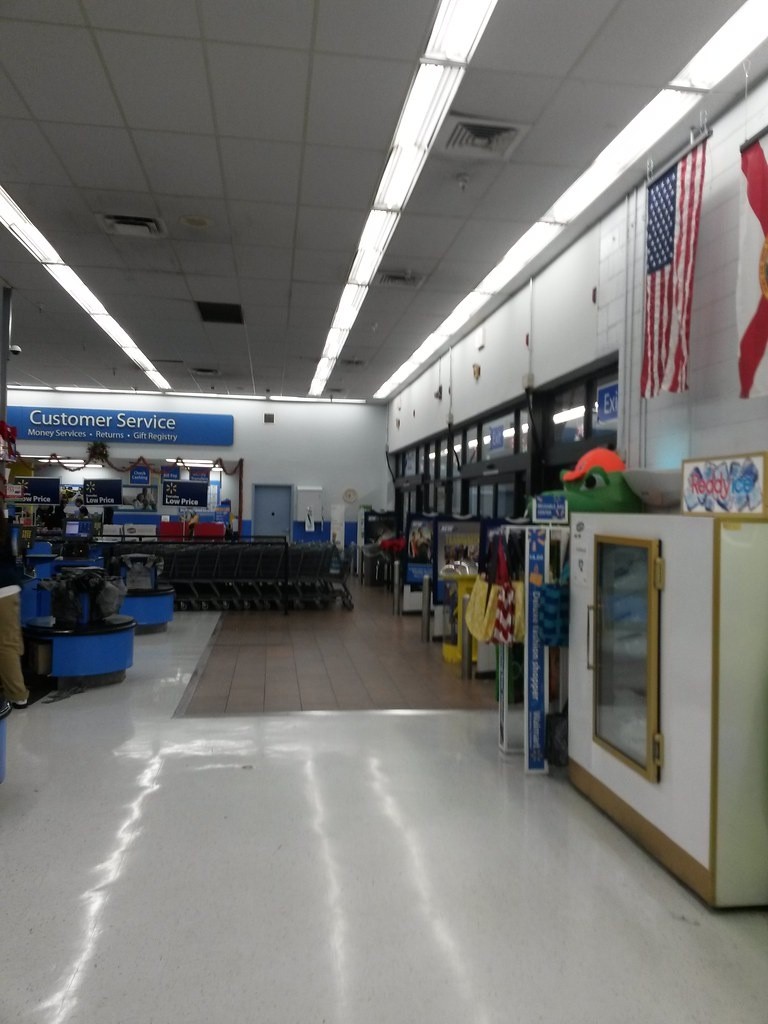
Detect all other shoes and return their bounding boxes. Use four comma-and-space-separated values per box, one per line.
9, 696, 28, 709
0, 700, 12, 718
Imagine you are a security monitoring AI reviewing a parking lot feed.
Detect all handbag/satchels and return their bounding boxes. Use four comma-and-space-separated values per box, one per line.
511, 532, 525, 645
537, 539, 569, 649
494, 534, 517, 647
464, 536, 501, 643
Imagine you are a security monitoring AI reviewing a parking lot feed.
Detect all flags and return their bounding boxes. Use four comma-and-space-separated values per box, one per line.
734, 127, 768, 399
640, 128, 711, 400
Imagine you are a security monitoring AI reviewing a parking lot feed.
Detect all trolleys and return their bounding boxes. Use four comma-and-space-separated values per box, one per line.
106, 540, 355, 612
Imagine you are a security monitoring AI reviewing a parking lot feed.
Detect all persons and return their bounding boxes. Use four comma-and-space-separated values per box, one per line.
35, 486, 89, 528
133, 487, 156, 510
